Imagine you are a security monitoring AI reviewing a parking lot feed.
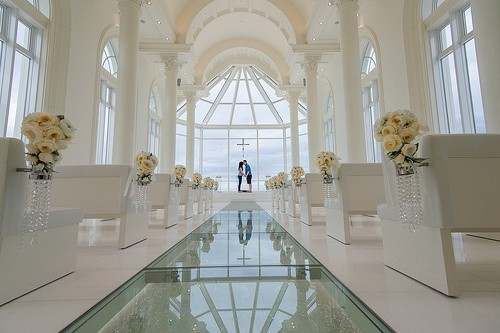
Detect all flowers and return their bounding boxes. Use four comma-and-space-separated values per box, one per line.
314, 150, 342, 184
264, 223, 312, 292
19, 112, 78, 176
202, 177, 220, 191
290, 166, 307, 188
135, 150, 159, 187
264, 171, 289, 190
192, 172, 202, 189
374, 108, 431, 176
161, 225, 219, 299
172, 164, 187, 187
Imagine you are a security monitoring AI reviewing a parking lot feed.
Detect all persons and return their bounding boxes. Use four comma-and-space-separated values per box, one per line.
243, 160, 252, 192
237, 210, 253, 245
238, 162, 246, 192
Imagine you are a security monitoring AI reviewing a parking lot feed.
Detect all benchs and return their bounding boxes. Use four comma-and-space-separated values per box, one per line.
210, 190, 217, 208
287, 178, 299, 219
280, 174, 289, 213
322, 162, 386, 246
276, 189, 280, 209
178, 178, 194, 220
272, 189, 276, 207
203, 190, 210, 211
298, 172, 325, 227
374, 132, 500, 299
52, 165, 151, 250
194, 189, 204, 214
0, 137, 83, 306
148, 174, 181, 228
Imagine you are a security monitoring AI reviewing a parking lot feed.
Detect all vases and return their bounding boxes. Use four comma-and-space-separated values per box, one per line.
323, 182, 336, 209
25, 176, 53, 233
393, 172, 425, 233
136, 185, 149, 215
